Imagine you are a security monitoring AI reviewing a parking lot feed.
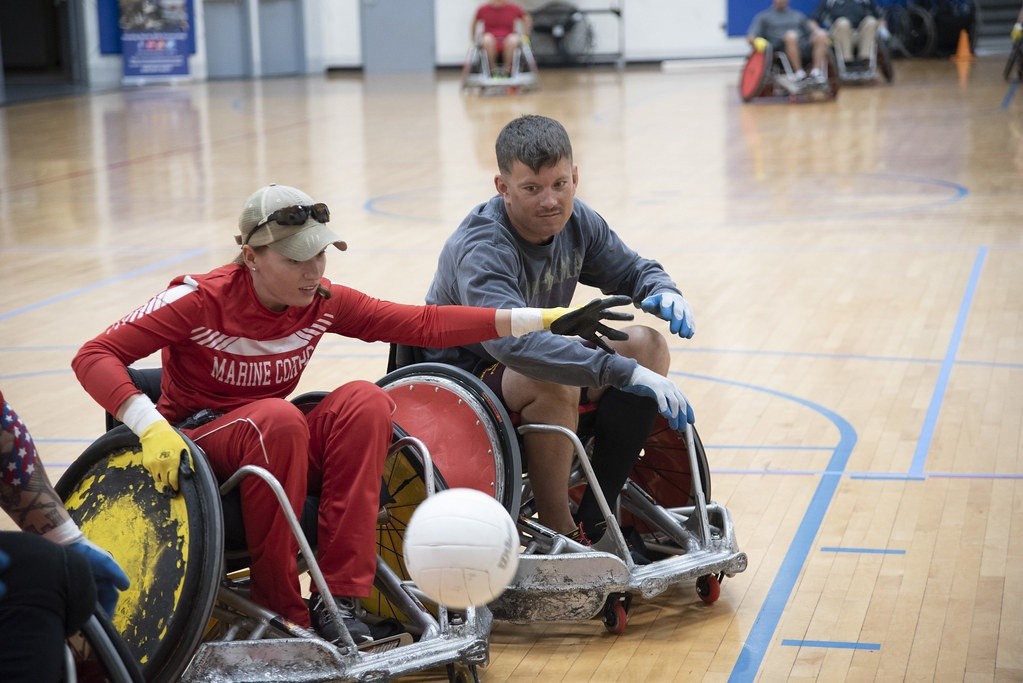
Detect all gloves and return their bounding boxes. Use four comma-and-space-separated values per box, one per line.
509, 295, 634, 355
641, 292, 695, 339
122, 393, 194, 496
39, 518, 130, 621
622, 363, 695, 432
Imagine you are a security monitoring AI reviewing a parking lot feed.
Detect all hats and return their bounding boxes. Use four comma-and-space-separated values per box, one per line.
234, 183, 347, 262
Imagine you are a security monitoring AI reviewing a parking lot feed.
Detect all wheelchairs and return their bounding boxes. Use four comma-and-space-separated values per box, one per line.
461, 33, 540, 96
736, 42, 841, 103
820, 25, 893, 84
371, 341, 720, 634
899, 3, 978, 55
49, 366, 494, 683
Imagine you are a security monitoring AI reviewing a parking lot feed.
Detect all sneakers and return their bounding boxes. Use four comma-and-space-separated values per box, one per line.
591, 521, 652, 566
308, 593, 375, 646
565, 521, 595, 549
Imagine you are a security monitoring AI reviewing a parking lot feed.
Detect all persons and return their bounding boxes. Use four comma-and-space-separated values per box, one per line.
0, 393, 129, 683
471, 0, 531, 79
72, 185, 634, 647
1011, 7, 1023, 45
815, 0, 886, 68
421, 115, 694, 562
746, 0, 831, 84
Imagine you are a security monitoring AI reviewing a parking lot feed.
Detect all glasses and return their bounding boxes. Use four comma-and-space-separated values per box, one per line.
241, 203, 331, 249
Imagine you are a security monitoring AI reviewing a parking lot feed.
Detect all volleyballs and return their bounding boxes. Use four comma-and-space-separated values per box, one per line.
401, 487, 521, 610
